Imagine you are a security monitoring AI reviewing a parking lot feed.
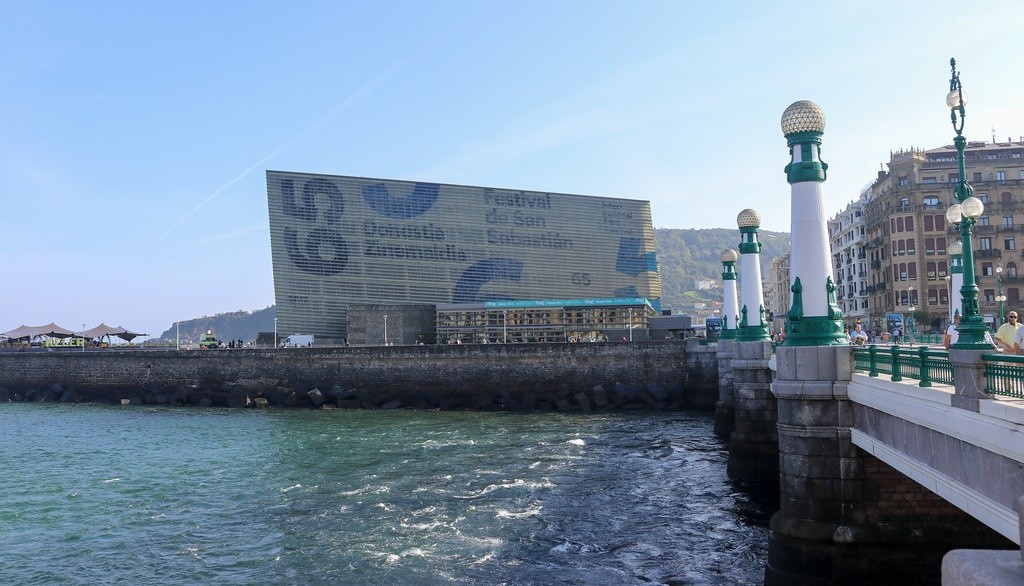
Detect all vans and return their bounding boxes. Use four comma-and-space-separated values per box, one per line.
199, 335, 218, 348
278, 333, 315, 348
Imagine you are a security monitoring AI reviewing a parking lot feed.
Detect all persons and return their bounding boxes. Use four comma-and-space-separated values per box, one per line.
994, 311, 1023, 397
944, 314, 961, 383
1013, 326, 1024, 356
0, 322, 903, 368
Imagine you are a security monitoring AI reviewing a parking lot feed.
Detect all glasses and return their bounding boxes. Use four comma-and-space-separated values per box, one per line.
1009, 316, 1018, 318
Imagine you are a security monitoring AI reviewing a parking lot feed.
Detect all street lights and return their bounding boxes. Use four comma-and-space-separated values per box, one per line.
996, 267, 1006, 326
273, 318, 277, 348
946, 58, 998, 350
383, 314, 388, 343
503, 310, 508, 343
82, 323, 86, 352
908, 284, 917, 334
628, 307, 633, 341
